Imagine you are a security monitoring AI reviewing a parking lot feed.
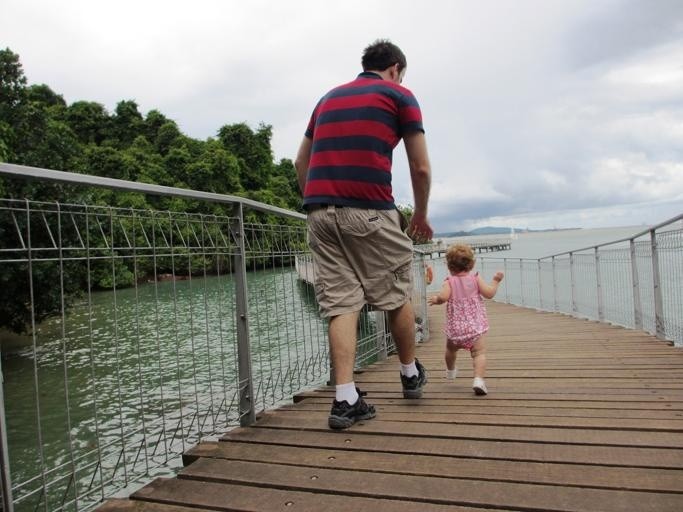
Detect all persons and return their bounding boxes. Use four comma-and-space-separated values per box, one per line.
426, 244, 504, 394
295, 38, 433, 430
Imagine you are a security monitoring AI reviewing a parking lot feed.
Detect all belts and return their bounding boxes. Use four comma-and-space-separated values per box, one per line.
305, 201, 343, 214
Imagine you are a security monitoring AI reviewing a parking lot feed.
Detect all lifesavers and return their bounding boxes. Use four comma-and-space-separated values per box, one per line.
425, 264, 433, 285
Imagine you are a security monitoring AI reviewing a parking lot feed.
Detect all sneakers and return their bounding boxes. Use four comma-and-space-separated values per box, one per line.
446, 369, 457, 383
471, 376, 489, 396
398, 357, 430, 401
327, 387, 377, 429
